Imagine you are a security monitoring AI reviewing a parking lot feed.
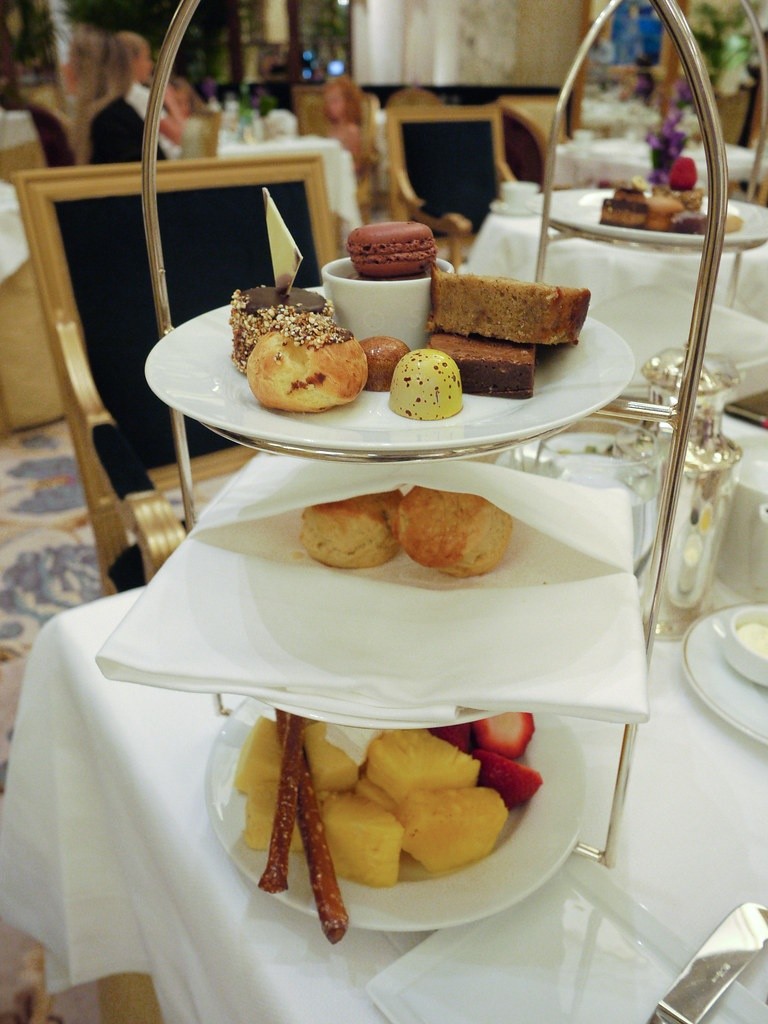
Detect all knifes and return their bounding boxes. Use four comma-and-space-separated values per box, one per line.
648, 902, 768, 1023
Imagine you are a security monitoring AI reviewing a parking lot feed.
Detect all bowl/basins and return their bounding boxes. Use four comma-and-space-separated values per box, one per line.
722, 605, 768, 687
499, 180, 537, 211
318, 257, 456, 351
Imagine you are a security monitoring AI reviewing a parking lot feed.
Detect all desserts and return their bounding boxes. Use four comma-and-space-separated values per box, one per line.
230, 186, 591, 421
600, 175, 743, 235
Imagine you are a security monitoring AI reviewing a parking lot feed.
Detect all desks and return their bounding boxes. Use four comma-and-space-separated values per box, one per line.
556, 140, 768, 205
462, 189, 768, 406
581, 97, 698, 142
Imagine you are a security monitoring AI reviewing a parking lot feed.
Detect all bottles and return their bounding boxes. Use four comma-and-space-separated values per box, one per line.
642, 345, 741, 640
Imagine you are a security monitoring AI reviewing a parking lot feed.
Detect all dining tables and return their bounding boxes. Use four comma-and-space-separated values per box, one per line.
0, 414, 768, 1024
0, 110, 365, 283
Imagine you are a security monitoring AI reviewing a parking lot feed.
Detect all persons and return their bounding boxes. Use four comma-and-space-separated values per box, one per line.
324, 76, 362, 177
117, 32, 185, 158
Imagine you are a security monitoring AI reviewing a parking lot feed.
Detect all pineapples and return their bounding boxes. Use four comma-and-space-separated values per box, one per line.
235, 716, 508, 888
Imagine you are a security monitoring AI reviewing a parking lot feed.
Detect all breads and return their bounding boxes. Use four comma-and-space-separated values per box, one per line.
301, 485, 512, 576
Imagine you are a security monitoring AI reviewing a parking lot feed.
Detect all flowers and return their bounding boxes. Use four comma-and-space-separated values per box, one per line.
646, 83, 693, 183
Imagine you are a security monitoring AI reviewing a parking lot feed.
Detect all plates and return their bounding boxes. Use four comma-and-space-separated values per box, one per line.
142, 284, 636, 450
207, 698, 588, 932
530, 189, 768, 246
247, 693, 504, 730
678, 602, 768, 745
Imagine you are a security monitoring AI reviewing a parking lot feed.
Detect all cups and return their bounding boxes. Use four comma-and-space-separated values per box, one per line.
512, 416, 663, 581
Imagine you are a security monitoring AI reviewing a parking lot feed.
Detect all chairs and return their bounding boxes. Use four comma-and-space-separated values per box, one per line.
15, 152, 344, 596
382, 104, 519, 273
497, 96, 582, 188
293, 85, 381, 222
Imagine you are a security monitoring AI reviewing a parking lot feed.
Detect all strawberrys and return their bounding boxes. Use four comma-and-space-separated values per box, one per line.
428, 712, 542, 811
669, 157, 697, 189
